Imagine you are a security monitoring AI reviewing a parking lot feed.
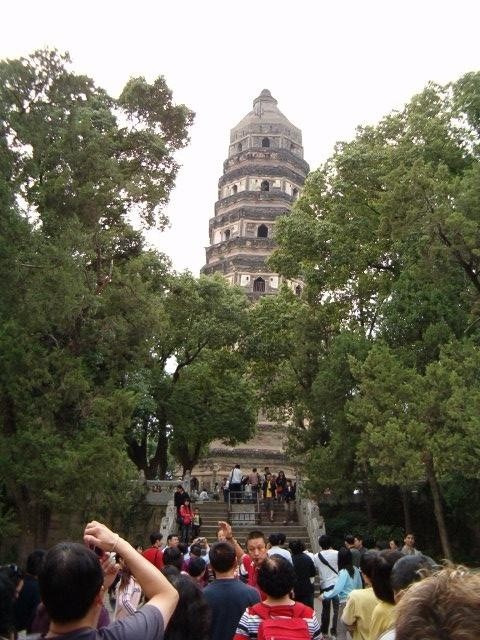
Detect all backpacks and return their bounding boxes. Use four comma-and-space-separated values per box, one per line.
251, 600, 314, 640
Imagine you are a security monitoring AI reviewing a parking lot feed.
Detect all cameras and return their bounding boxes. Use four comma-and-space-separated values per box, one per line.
199, 537, 205, 542
